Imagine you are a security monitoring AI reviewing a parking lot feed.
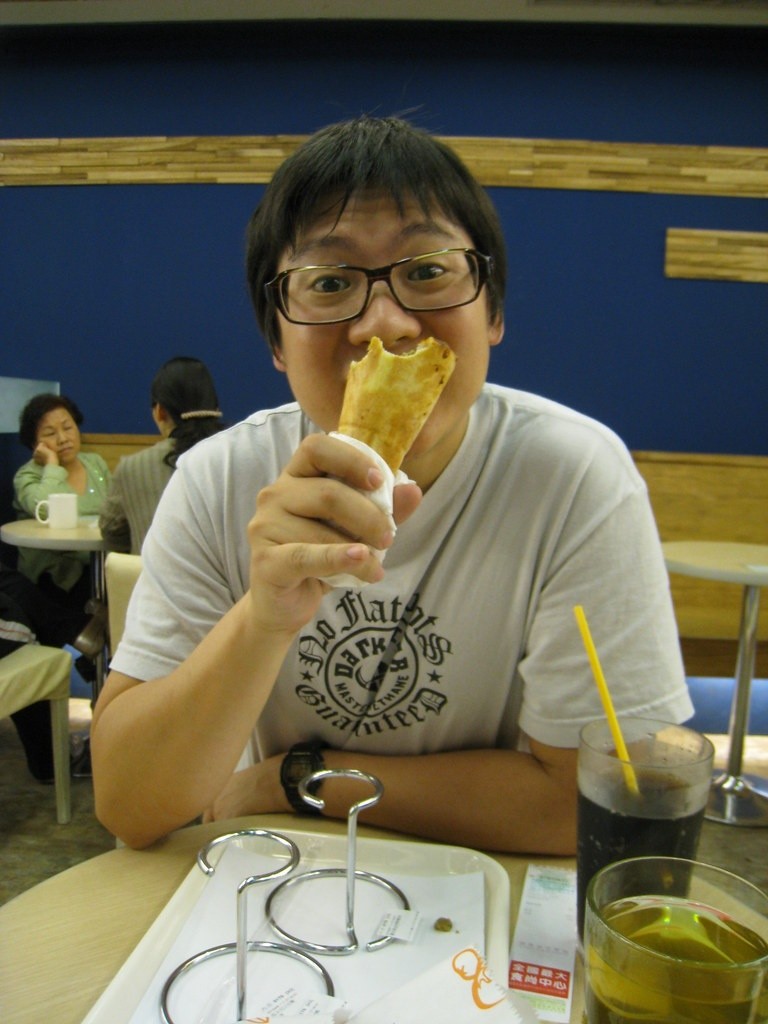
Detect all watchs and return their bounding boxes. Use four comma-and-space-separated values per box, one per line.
280, 741, 331, 817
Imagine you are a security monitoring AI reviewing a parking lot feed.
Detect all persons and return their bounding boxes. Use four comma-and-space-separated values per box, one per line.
12, 393, 110, 618
0, 594, 85, 783
93, 118, 697, 851
99, 356, 230, 554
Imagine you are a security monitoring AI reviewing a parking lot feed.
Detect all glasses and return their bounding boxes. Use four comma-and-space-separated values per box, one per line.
262, 247, 492, 326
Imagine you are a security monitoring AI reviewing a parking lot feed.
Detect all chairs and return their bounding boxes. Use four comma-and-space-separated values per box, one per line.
0, 644, 72, 825
103, 551, 142, 660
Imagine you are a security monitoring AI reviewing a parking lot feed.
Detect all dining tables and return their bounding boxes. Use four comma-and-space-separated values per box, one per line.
660, 540, 768, 827
0, 515, 110, 781
0, 810, 768, 1024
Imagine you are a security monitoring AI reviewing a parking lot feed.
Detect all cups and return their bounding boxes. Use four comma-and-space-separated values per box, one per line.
570, 719, 715, 1024
36, 494, 77, 529
583, 857, 768, 1024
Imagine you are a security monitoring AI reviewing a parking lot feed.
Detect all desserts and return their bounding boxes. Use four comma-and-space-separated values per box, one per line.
337, 336, 456, 479
